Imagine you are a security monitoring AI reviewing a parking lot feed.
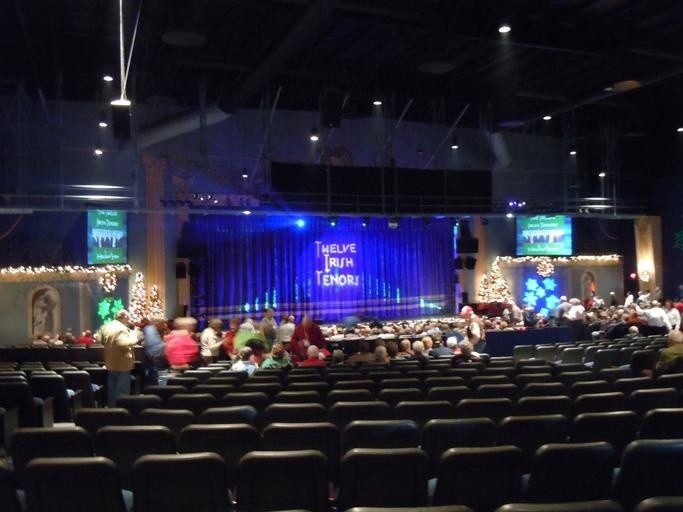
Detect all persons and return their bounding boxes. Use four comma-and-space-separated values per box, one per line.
321, 304, 483, 365
95, 308, 143, 403
643, 330, 683, 377
480, 290, 683, 340
126, 307, 331, 378
36, 327, 99, 347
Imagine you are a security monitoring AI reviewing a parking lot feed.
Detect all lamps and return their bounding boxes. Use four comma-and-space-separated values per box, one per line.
241, 94, 460, 226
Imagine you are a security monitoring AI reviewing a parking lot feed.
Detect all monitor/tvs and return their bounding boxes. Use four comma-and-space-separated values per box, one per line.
85, 209, 128, 266
514, 215, 574, 259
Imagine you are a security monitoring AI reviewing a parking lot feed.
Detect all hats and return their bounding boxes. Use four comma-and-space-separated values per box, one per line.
459, 305, 472, 318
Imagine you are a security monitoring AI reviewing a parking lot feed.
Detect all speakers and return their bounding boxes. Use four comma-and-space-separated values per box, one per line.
457, 237, 479, 253
453, 256, 463, 269
464, 256, 476, 269
